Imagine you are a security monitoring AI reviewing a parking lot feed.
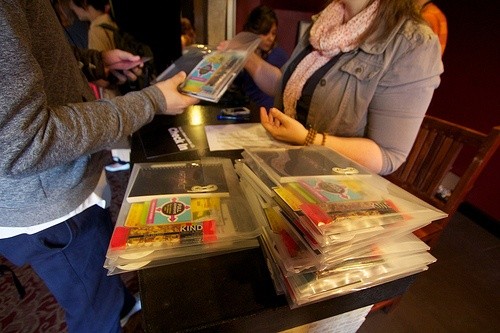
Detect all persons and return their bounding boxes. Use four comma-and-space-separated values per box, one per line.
234, 6, 289, 113
69, 0, 131, 172
181, 17, 196, 49
407, 0, 448, 49
244, 0, 445, 176
0, 0, 201, 333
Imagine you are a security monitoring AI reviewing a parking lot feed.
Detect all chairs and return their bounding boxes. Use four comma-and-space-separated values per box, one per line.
364, 115, 500, 319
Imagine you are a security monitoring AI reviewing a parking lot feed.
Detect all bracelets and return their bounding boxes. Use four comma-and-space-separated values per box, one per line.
304, 128, 326, 146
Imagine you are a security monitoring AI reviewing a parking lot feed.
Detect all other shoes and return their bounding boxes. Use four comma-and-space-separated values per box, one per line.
117, 292, 141, 328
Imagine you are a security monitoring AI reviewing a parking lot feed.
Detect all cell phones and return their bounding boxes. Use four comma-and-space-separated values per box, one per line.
222, 107, 250, 115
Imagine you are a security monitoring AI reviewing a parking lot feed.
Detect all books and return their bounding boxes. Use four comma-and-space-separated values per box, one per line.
150, 39, 261, 103
104, 146, 449, 309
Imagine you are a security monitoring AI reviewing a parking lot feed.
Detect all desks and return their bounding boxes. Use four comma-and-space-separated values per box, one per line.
136, 93, 419, 333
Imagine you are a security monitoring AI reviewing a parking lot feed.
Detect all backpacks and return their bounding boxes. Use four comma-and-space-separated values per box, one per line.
97, 21, 154, 96
